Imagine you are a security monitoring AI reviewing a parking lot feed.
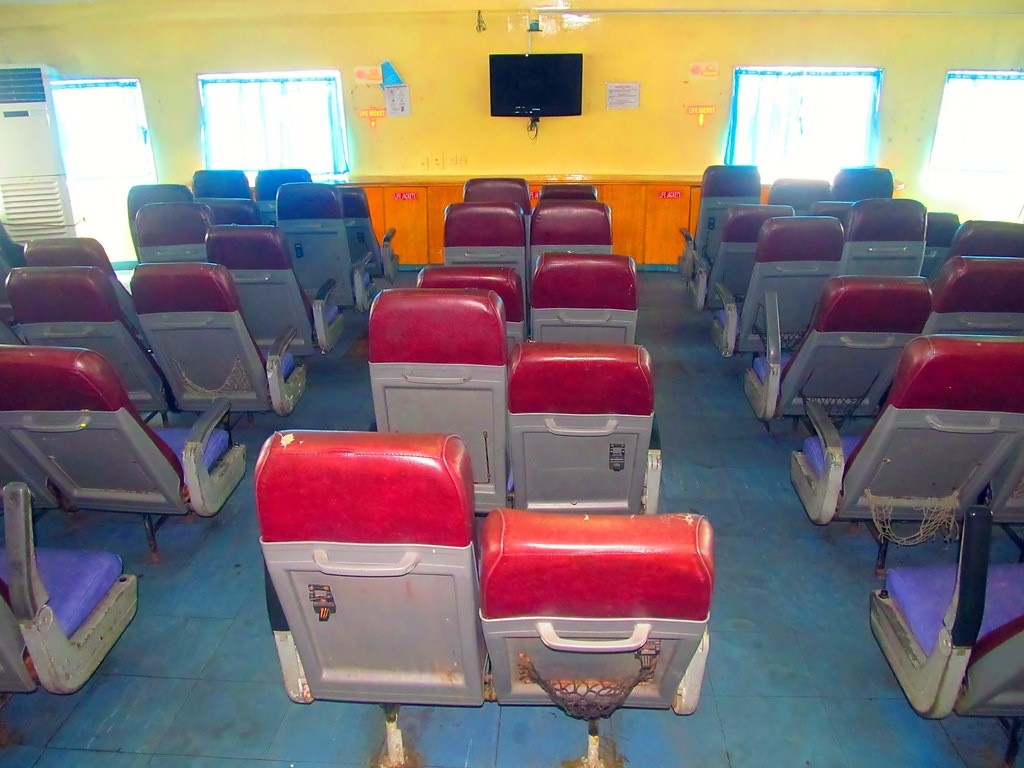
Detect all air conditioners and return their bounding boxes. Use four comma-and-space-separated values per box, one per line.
0, 63, 77, 247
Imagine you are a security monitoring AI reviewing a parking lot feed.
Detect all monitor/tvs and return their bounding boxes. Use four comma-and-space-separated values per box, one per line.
489, 54, 582, 117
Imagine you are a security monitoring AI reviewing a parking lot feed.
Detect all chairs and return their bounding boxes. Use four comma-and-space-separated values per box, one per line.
0, 167, 1024, 768
